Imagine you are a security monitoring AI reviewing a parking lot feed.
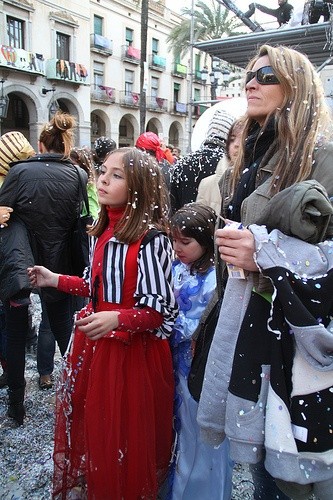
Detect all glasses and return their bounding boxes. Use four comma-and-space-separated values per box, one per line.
246, 66, 279, 85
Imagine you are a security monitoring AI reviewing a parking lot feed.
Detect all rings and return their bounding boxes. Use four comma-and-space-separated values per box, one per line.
191, 347, 194, 350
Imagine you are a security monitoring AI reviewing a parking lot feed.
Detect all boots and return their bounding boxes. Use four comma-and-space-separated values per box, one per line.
6, 387, 26, 426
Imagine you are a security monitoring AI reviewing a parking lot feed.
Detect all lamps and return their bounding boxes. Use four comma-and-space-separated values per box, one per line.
0, 77, 10, 123
39, 81, 60, 121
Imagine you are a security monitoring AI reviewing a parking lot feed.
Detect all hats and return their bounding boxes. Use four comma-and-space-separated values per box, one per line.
94, 136, 116, 159
207, 109, 236, 140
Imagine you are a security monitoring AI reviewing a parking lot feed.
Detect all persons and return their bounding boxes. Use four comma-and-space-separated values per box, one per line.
55, 60, 88, 82
18, 50, 46, 72
0, 111, 88, 426
136, 131, 174, 193
170, 109, 237, 218
161, 143, 182, 164
0, 114, 89, 388
27, 148, 180, 500
0, 131, 56, 388
169, 202, 232, 500
196, 118, 245, 215
92, 136, 116, 174
187, 45, 333, 500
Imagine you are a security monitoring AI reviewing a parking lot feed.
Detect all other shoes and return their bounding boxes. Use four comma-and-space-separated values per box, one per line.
39, 375, 53, 391
